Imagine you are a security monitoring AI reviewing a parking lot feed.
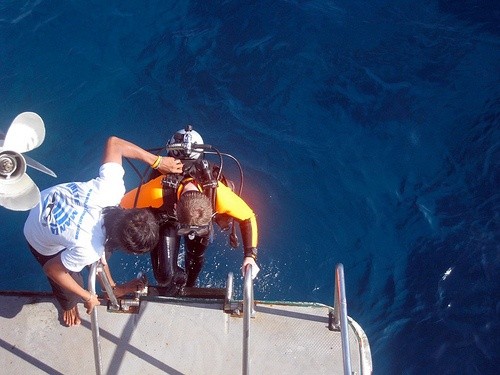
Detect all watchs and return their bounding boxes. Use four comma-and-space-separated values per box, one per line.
245, 247, 258, 256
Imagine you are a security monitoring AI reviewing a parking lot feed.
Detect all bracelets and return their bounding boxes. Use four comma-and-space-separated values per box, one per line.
151, 156, 162, 169
81, 291, 92, 302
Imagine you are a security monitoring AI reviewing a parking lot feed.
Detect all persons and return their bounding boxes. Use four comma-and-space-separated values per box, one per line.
120, 173, 260, 287
23, 136, 183, 327
